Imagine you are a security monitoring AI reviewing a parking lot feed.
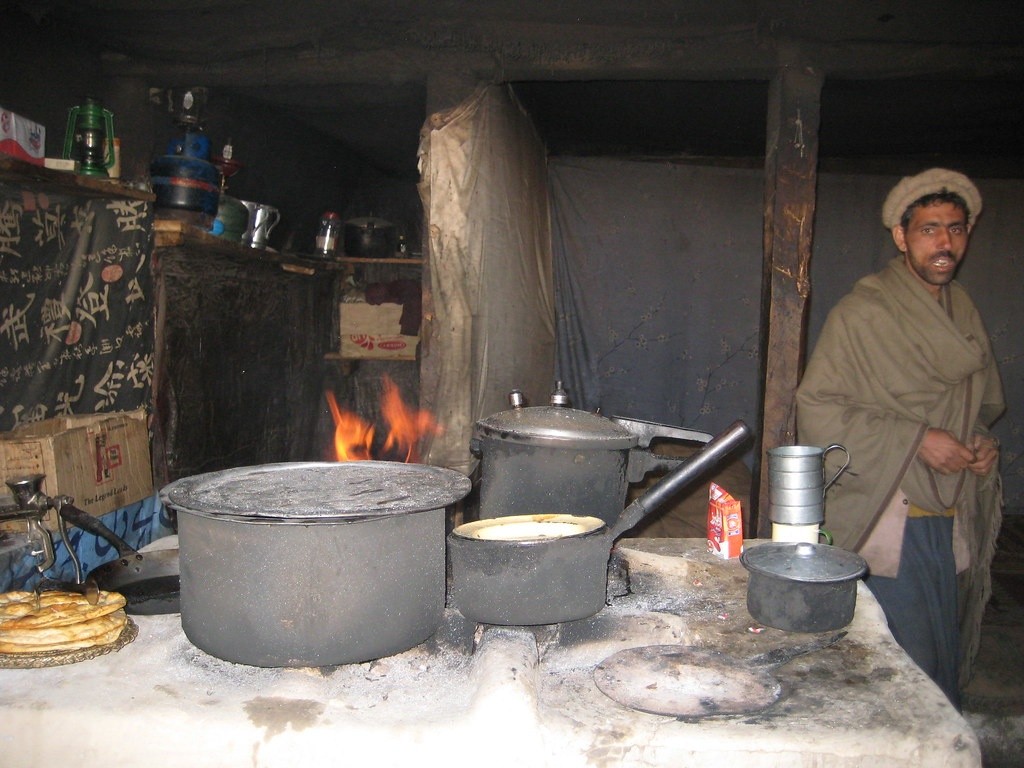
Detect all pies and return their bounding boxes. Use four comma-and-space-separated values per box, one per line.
0, 589, 128, 653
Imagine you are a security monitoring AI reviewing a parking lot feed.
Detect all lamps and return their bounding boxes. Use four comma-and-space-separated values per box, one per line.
62, 95, 116, 179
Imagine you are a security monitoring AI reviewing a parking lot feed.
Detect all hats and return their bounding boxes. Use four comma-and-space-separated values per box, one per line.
881, 167, 982, 234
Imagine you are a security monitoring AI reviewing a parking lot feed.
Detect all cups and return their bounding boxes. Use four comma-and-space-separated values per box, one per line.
771, 522, 834, 547
765, 440, 850, 526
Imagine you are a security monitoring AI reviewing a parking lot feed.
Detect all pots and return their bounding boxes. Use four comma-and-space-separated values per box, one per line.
58, 500, 180, 617
446, 418, 753, 627
468, 378, 715, 532
739, 543, 869, 633
158, 459, 472, 668
345, 210, 400, 257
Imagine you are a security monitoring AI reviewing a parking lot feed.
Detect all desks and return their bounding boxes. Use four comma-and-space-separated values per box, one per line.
151, 223, 323, 483
0, 537, 982, 768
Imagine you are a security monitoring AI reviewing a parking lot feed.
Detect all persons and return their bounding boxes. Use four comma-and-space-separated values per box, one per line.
796, 169, 1006, 716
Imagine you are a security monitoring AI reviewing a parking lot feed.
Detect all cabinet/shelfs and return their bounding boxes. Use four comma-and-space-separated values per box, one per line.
324, 254, 423, 362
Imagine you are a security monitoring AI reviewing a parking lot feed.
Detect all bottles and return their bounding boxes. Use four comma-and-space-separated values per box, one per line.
314, 210, 342, 259
400, 235, 408, 257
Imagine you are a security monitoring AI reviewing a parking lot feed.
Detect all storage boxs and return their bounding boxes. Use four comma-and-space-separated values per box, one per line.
0, 408, 155, 533
